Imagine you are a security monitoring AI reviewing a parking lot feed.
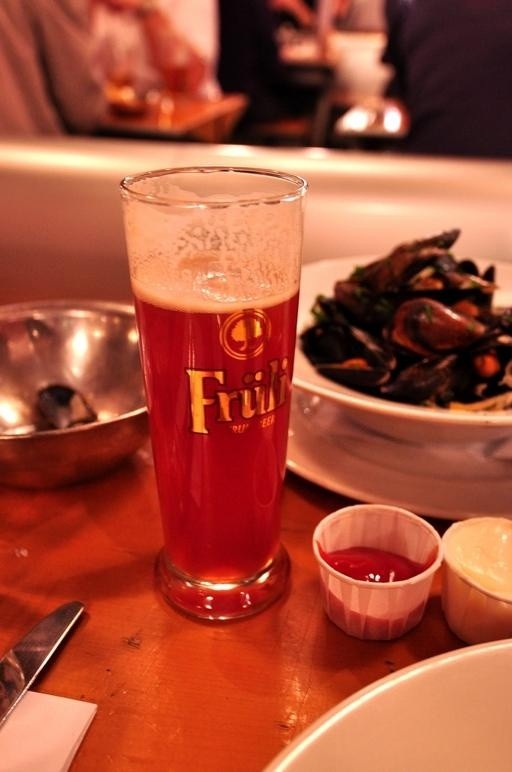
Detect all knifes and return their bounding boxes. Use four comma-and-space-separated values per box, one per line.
0, 599, 86, 730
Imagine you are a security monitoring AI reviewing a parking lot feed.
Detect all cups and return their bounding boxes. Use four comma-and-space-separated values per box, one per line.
114, 161, 311, 626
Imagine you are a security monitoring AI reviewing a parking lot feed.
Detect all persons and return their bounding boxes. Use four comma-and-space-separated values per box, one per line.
2, 2, 512, 164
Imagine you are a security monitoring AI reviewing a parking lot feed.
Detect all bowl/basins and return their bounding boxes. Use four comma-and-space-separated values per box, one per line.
291, 251, 512, 449
0, 295, 152, 491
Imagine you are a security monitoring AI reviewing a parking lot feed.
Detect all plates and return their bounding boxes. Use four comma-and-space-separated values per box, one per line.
285, 387, 512, 521
